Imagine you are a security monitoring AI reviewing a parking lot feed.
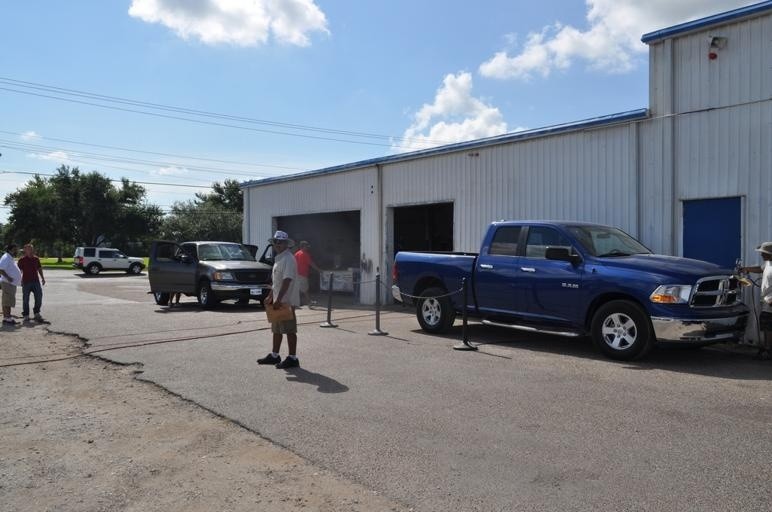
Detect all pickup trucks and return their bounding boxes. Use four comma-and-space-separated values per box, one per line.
390, 218, 751, 360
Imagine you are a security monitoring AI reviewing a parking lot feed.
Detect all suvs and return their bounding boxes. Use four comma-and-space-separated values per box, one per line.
73, 248, 146, 276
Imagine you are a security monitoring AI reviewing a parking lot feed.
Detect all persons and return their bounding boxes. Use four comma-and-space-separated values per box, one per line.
294, 240, 326, 305
0, 245, 22, 325
736, 241, 771, 357
168, 291, 182, 306
255, 229, 301, 370
18, 244, 46, 322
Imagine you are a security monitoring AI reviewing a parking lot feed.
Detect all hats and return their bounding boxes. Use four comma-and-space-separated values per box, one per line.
754, 241, 772, 256
267, 230, 296, 248
300, 240, 311, 248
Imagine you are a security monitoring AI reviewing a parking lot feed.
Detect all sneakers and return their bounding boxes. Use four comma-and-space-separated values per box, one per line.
274, 355, 299, 369
23, 315, 29, 322
256, 353, 281, 364
33, 313, 50, 324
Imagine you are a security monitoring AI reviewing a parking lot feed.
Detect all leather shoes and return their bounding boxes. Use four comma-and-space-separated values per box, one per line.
2, 318, 22, 327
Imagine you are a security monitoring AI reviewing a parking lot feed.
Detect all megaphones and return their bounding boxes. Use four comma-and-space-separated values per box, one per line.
707, 36, 728, 48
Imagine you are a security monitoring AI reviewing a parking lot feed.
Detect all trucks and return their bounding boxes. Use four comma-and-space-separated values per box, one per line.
147, 238, 274, 307
